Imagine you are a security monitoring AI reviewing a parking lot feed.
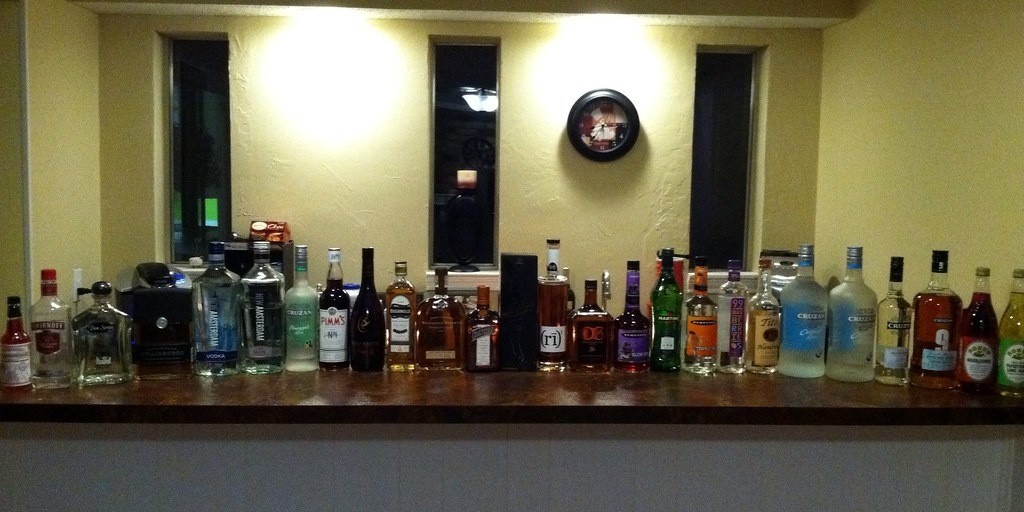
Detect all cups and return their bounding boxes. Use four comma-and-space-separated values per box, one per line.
252, 222, 283, 241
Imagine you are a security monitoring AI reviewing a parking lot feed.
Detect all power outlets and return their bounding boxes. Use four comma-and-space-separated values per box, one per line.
72, 269, 82, 302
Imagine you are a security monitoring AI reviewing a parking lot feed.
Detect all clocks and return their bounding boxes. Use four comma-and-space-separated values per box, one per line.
567, 89, 640, 161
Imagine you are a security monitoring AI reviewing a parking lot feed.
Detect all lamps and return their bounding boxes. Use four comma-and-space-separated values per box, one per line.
457, 170, 477, 189
457, 86, 498, 113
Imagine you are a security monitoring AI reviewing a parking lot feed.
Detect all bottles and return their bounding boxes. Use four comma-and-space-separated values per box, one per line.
385, 261, 417, 373
824, 246, 877, 382
318, 248, 350, 372
28, 269, 74, 390
680, 256, 718, 374
0, 296, 30, 392
777, 245, 827, 378
466, 284, 500, 373
350, 247, 386, 373
717, 260, 749, 375
743, 259, 779, 374
416, 268, 466, 370
238, 241, 287, 374
71, 281, 134, 388
908, 249, 1024, 398
537, 238, 683, 374
191, 241, 240, 376
284, 245, 319, 372
874, 256, 912, 386
